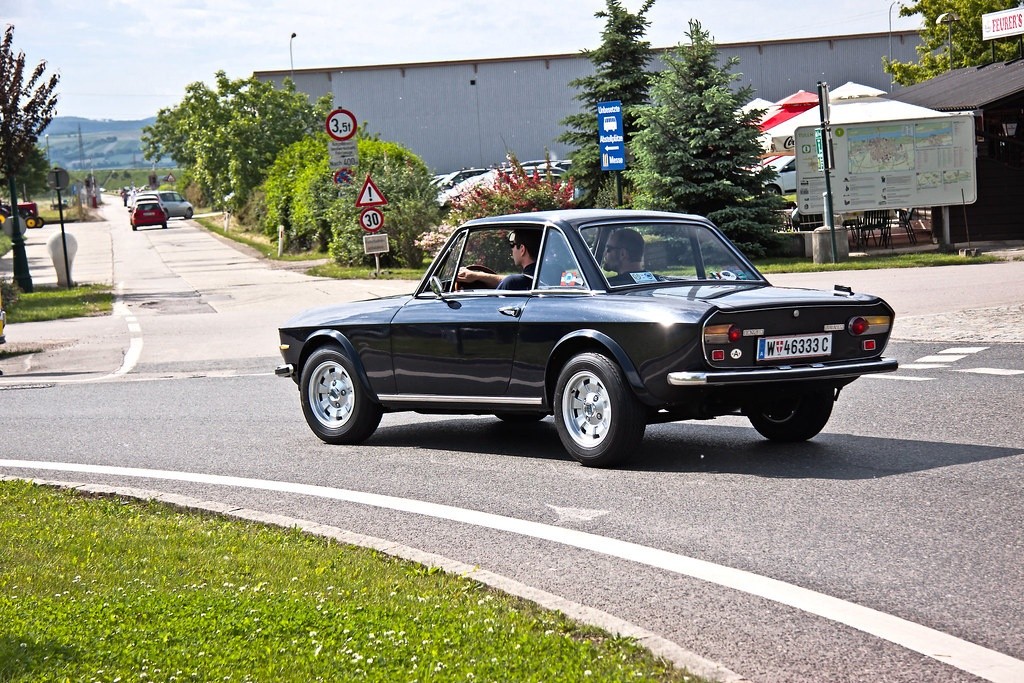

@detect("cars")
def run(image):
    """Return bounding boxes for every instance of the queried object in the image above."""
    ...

[273,208,899,466]
[130,200,168,230]
[132,197,162,213]
[425,159,583,208]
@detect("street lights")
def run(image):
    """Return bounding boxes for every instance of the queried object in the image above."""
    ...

[289,34,296,81]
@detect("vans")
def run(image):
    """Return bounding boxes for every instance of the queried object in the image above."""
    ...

[141,191,194,219]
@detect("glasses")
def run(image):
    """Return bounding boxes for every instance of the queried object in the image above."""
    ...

[509,241,515,248]
[605,245,621,252]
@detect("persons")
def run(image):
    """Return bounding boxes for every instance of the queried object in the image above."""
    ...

[603,228,668,286]
[455,228,562,297]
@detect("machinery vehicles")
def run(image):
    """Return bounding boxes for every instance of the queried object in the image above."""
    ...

[0,200,44,228]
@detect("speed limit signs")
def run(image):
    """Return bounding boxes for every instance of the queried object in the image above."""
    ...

[360,208,384,232]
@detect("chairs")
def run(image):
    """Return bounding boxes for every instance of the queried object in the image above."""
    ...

[841,207,920,253]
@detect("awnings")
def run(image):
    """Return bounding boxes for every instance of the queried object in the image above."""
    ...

[737,79,952,152]
